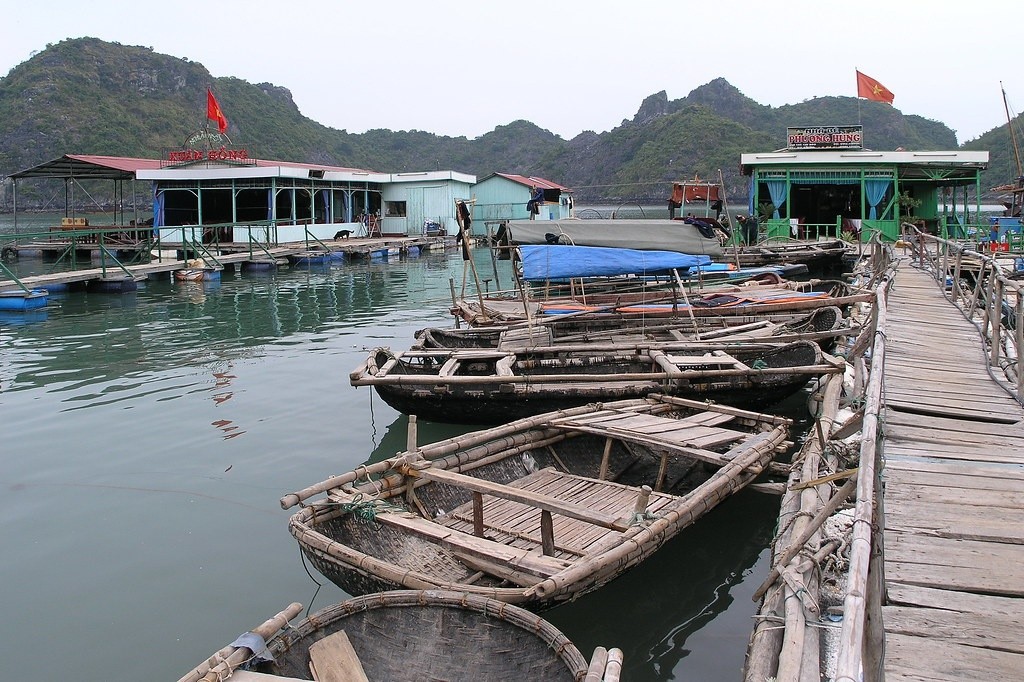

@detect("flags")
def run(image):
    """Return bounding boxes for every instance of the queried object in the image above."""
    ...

[207,88,228,133]
[856,70,895,105]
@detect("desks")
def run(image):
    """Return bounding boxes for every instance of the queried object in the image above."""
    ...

[48,223,154,243]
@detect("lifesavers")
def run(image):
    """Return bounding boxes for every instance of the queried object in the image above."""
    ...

[1,246,18,259]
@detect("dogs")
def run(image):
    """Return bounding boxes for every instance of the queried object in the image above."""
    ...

[333,230,355,242]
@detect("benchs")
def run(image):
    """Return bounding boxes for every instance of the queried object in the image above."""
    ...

[404,466,630,558]
[495,356,516,377]
[375,350,406,377]
[333,487,570,579]
[439,358,461,377]
[543,409,753,465]
[650,350,749,373]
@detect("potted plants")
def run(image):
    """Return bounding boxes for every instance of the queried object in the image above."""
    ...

[893,191,923,243]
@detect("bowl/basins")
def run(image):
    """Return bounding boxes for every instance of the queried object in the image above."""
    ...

[427,231,439,237]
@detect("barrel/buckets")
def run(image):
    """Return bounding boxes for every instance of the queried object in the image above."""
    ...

[1014,258,1024,274]
[424,221,440,234]
[439,230,447,236]
[976,240,1009,253]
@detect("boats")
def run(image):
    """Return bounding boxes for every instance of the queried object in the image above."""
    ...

[278,395,797,616]
[410,172,857,356]
[176,591,625,682]
[350,340,848,424]
